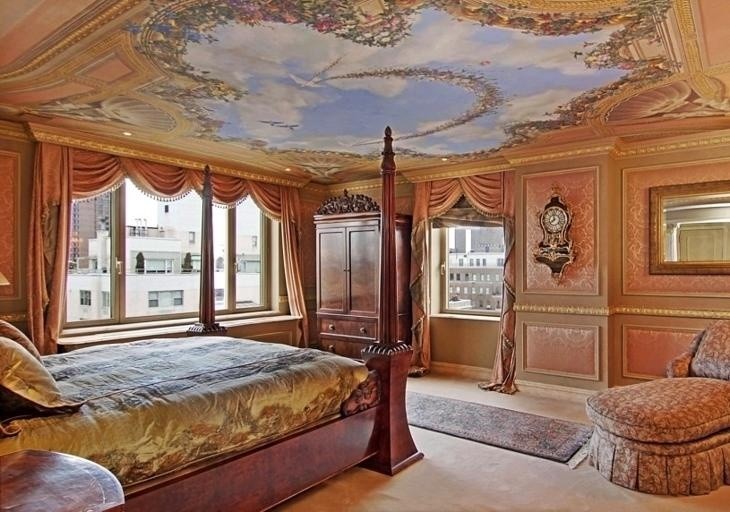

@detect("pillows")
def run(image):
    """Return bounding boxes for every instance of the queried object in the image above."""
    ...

[1,318,88,436]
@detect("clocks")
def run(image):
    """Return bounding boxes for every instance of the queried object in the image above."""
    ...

[533,195,578,287]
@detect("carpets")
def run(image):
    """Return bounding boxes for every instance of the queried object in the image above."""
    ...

[405,391,594,471]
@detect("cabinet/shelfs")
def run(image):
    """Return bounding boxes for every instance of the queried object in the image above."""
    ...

[311,188,413,359]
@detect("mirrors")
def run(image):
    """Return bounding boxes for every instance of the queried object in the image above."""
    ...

[649,179,730,275]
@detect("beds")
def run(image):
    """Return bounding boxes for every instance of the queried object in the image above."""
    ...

[2,336,424,511]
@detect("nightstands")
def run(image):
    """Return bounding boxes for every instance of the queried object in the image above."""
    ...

[0,449,125,512]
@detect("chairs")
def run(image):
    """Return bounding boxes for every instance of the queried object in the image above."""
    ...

[585,318,730,497]
